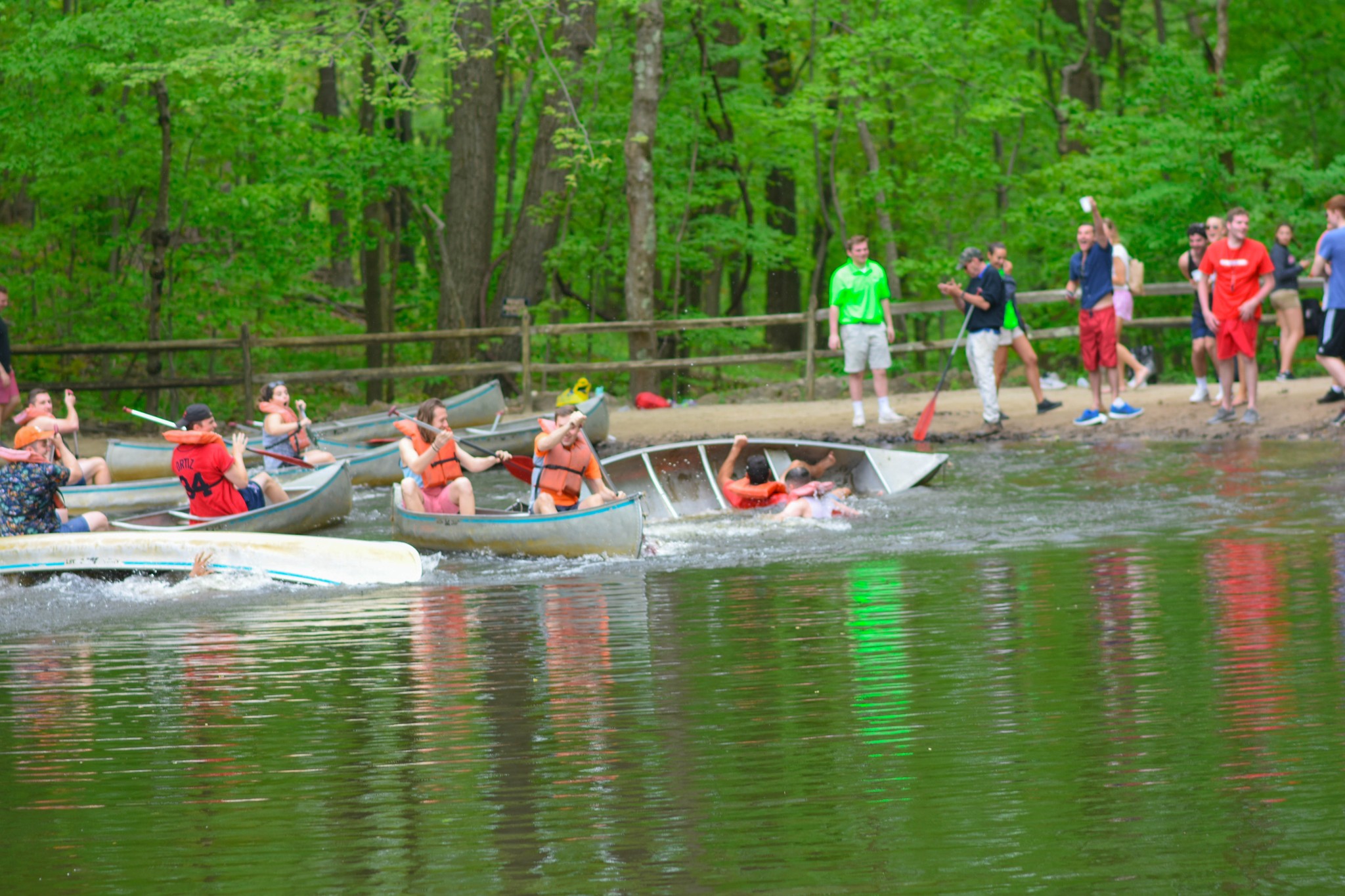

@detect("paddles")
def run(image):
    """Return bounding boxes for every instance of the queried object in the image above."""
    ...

[68,389,80,459]
[579,424,662,559]
[912,288,984,441]
[228,421,261,435]
[122,406,315,469]
[246,418,263,428]
[387,405,536,485]
[491,410,503,430]
[298,402,319,448]
[49,424,59,463]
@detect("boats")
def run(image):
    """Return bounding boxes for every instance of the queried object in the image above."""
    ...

[388,479,646,562]
[526,438,951,528]
[0,378,612,589]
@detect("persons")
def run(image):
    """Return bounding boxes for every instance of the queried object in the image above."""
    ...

[712,433,870,525]
[397,398,513,516]
[1309,194,1345,427]
[1198,207,1275,425]
[1063,196,1144,427]
[171,404,290,525]
[1097,217,1151,393]
[1267,222,1312,382]
[0,284,21,448]
[828,235,908,428]
[937,247,1006,437]
[0,423,111,540]
[13,387,111,486]
[1179,217,1249,406]
[181,549,214,583]
[528,404,626,515]
[257,381,336,470]
[984,241,1062,419]
[657,330,691,404]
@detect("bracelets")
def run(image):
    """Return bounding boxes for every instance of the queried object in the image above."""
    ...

[297,420,302,429]
[431,442,440,452]
[960,291,966,299]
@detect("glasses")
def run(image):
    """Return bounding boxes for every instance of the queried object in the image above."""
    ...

[268,380,285,387]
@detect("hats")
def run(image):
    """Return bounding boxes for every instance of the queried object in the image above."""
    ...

[13,426,55,449]
[175,404,214,428]
[956,247,984,270]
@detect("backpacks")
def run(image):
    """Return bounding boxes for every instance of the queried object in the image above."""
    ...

[1130,258,1144,295]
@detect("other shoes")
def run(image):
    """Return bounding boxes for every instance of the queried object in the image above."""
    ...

[1276,370,1297,381]
[1036,398,1062,414]
[1189,387,1210,403]
[1211,401,1224,406]
[1240,409,1262,425]
[877,411,910,424]
[851,414,866,428]
[977,419,1004,438]
[1216,388,1233,400]
[999,411,1010,420]
[1207,407,1236,424]
[1231,398,1249,405]
[1073,409,1107,426]
[1108,403,1144,420]
[1317,386,1345,404]
